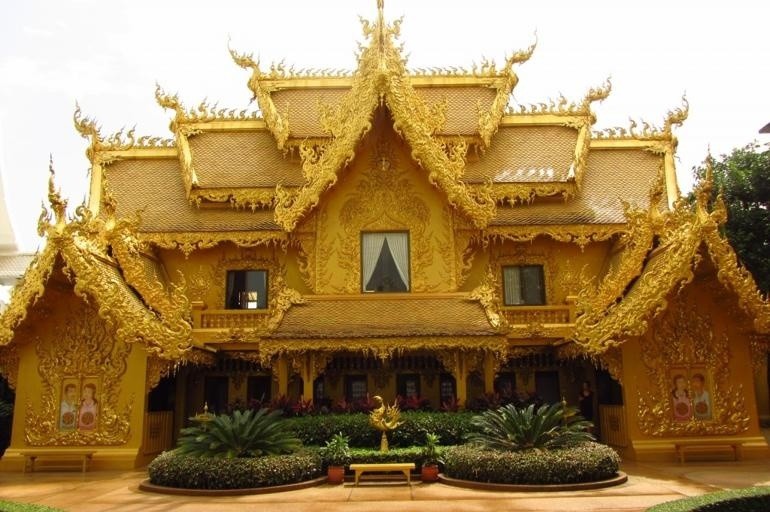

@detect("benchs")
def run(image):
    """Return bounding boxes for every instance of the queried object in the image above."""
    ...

[670,440,745,463]
[350,463,417,486]
[20,451,94,474]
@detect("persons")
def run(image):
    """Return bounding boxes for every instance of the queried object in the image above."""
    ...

[79,383,99,430]
[691,372,713,421]
[670,373,693,422]
[60,382,76,428]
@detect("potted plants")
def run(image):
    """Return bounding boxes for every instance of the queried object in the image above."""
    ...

[324,432,349,485]
[421,431,442,482]
